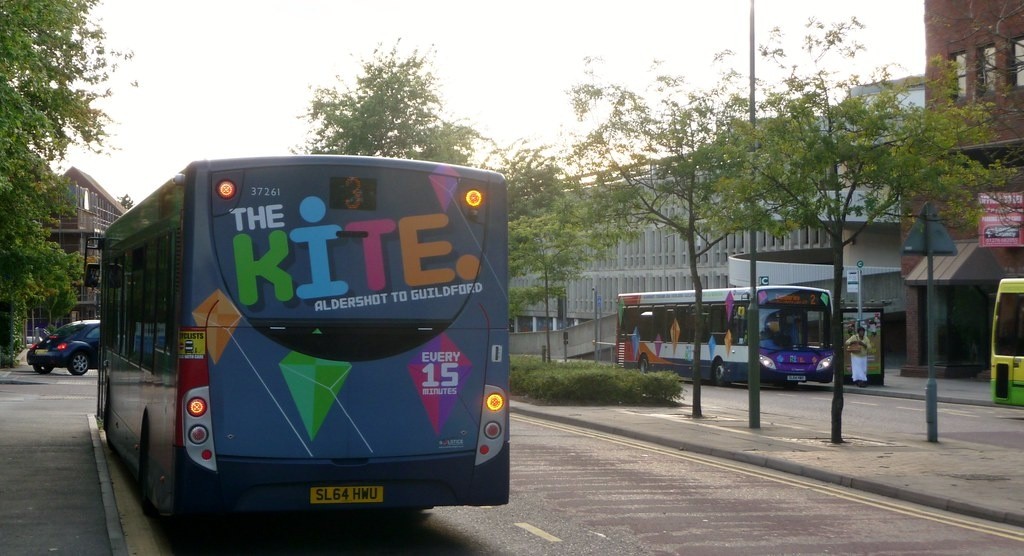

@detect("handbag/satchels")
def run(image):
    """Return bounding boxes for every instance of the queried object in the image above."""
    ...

[846,334,861,352]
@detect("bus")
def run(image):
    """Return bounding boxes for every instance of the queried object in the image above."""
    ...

[991,278,1024,407]
[615,286,860,386]
[85,155,511,523]
[27,330,50,344]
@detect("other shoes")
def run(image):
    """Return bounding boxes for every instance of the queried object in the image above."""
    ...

[855,380,868,388]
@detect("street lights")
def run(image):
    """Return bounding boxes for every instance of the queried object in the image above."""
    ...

[592,287,597,361]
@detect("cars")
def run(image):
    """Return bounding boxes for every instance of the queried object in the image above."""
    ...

[25,320,102,376]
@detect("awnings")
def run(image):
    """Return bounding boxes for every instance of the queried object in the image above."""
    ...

[904,238,1002,285]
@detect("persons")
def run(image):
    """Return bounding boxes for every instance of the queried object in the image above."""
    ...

[845,327,871,388]
